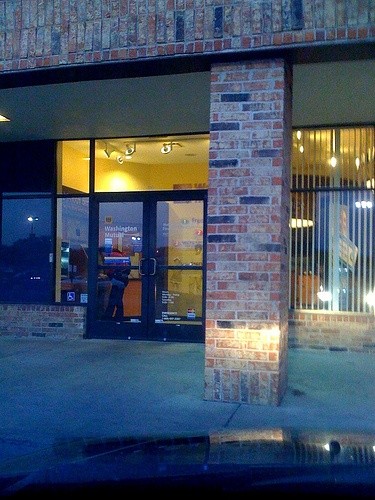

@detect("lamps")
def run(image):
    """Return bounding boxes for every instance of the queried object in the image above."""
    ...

[103,149,116,158]
[116,154,125,165]
[160,142,183,154]
[125,143,136,156]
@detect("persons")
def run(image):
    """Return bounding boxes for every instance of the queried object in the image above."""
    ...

[99,243,131,321]
